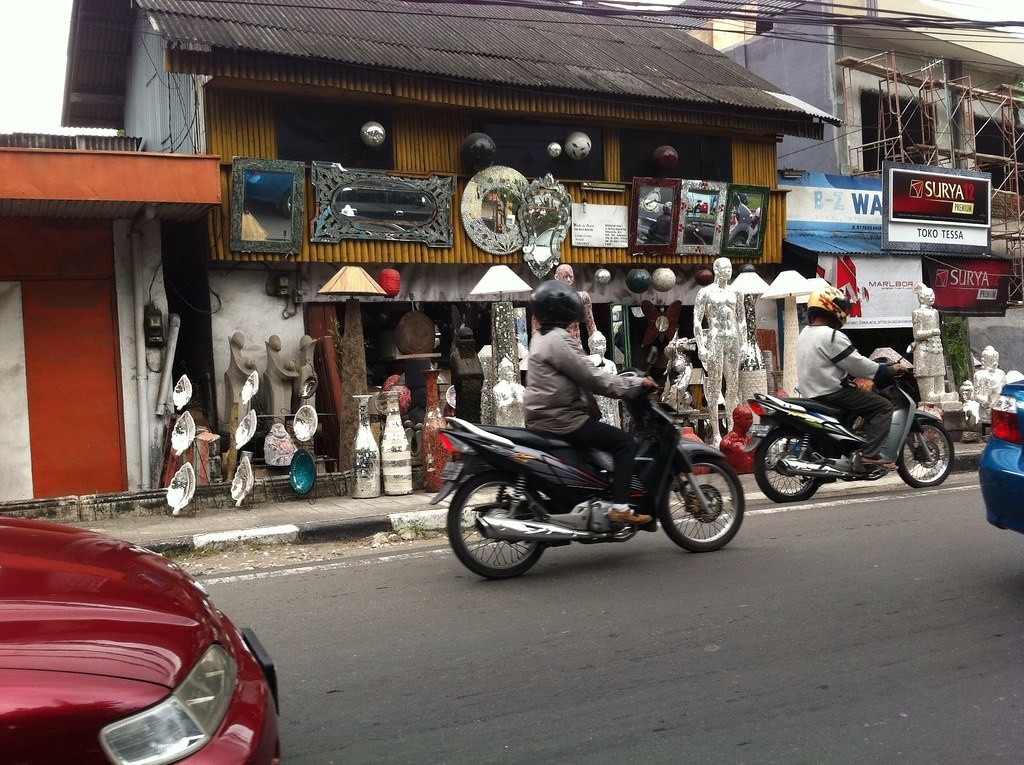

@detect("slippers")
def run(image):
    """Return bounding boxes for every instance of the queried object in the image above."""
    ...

[861,460,899,471]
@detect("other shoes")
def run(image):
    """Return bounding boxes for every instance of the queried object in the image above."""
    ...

[609,508,652,525]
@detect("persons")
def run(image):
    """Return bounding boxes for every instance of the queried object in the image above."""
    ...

[694,199,708,213]
[746,207,760,247]
[532,265,597,349]
[795,286,908,471]
[720,406,756,473]
[912,282,959,401]
[643,200,672,243]
[974,345,1006,423]
[694,257,747,449]
[493,357,526,429]
[588,331,621,429]
[524,280,659,523]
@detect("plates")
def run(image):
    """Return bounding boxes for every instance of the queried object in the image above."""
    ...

[300,376,317,399]
[289,449,316,494]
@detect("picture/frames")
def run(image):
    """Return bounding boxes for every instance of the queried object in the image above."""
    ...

[676,181,729,257]
[721,182,770,260]
[309,160,455,248]
[516,173,572,279]
[626,175,683,256]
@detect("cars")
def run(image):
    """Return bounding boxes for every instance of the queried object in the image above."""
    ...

[0,513,283,765]
[977,379,1024,536]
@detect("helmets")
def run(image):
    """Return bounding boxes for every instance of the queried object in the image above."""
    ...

[733,193,740,205]
[808,286,850,329]
[738,192,747,204]
[531,279,584,323]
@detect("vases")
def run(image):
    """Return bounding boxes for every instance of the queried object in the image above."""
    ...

[350,390,413,499]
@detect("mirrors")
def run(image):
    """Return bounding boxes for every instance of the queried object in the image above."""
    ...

[231,156,305,254]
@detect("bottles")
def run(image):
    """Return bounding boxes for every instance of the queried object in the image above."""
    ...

[292,405,317,441]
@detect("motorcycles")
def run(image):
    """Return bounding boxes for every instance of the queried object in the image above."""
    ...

[745,342,952,503]
[429,345,747,581]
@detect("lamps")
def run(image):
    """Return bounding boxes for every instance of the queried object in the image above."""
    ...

[467,265,534,305]
[317,263,387,300]
